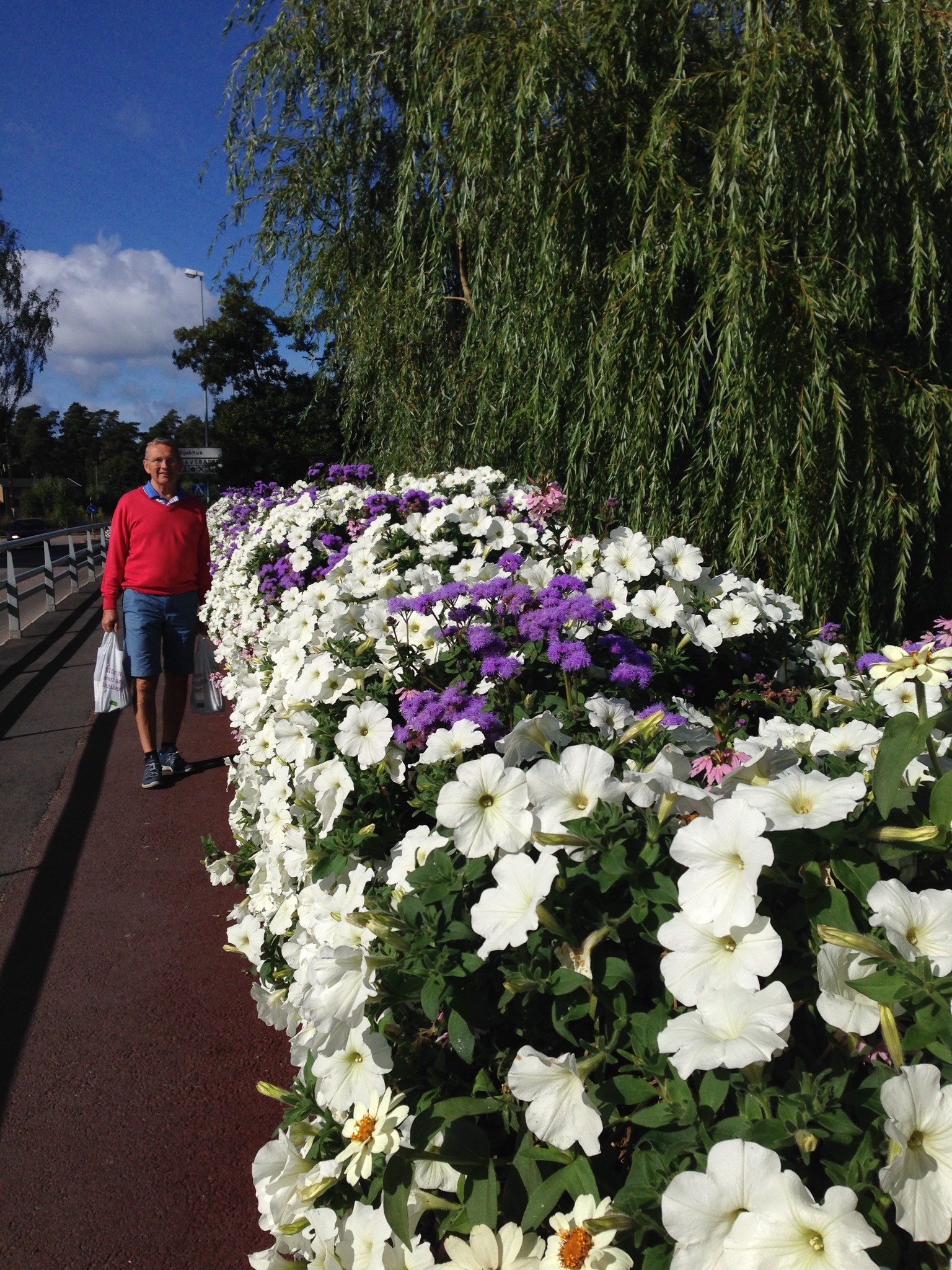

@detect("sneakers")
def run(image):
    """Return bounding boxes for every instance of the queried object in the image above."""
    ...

[158,747,194,773]
[142,755,164,788]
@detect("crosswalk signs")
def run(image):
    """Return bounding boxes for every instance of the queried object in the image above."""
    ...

[191,485,205,498]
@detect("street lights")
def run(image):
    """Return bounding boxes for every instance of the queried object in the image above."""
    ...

[185,269,210,509]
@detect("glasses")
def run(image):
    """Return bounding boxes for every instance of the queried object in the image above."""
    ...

[144,457,180,466]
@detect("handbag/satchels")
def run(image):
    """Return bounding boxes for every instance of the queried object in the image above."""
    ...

[93,630,132,714]
[190,632,225,715]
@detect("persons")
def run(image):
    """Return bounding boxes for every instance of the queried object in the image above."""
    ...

[101,438,211,788]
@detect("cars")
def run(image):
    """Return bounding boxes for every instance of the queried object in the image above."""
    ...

[103,526,111,539]
[7,518,51,548]
